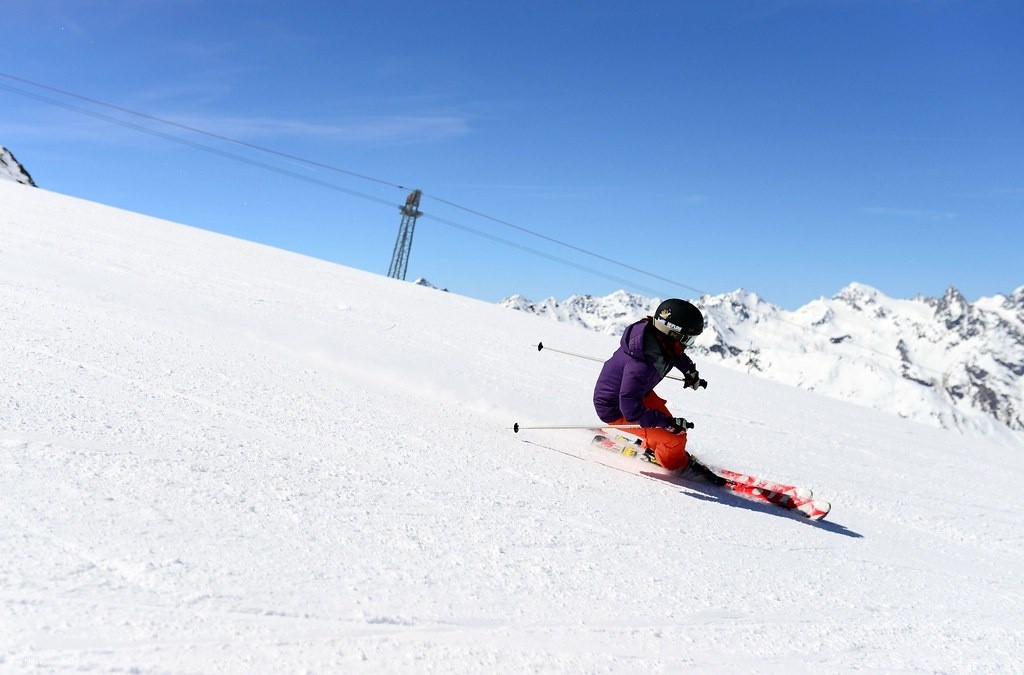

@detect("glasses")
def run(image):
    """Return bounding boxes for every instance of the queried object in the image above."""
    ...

[653,316,698,347]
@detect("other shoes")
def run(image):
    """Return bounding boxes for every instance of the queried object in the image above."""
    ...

[682,460,712,481]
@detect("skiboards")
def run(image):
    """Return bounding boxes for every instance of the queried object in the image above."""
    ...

[587,424,831,520]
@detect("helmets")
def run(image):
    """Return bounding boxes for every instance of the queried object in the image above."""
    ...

[654,299,703,344]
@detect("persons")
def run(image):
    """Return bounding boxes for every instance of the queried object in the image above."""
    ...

[593,299,722,486]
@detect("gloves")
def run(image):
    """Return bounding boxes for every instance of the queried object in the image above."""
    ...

[664,418,688,435]
[684,364,700,391]
